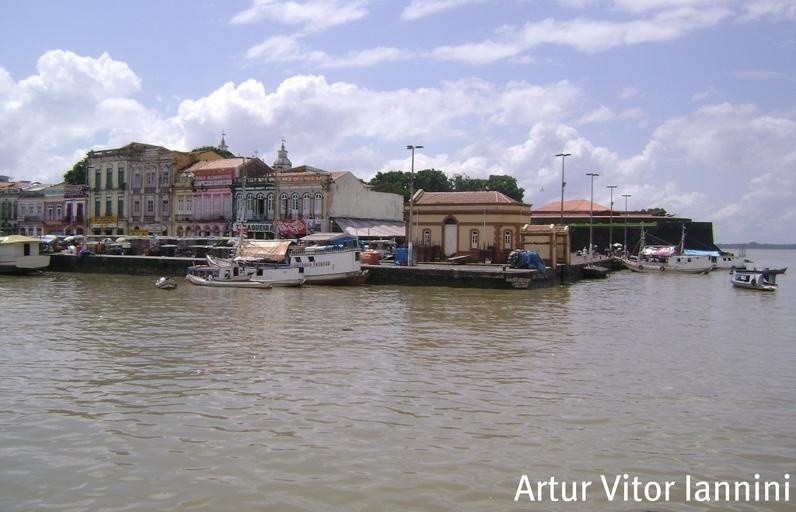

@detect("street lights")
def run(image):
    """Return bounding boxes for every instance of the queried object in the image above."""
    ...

[555,153,571,224]
[406,145,424,267]
[585,173,632,267]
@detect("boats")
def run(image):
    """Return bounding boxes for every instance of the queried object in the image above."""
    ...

[154,276,178,289]
[729,266,788,291]
[0,234,51,276]
[185,238,363,289]
[614,223,747,273]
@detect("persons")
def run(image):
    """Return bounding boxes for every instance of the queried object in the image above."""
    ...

[159,275,165,283]
[208,272,214,280]
[165,274,170,281]
[582,244,632,261]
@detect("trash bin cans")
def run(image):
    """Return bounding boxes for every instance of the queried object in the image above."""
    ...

[396,248,408,265]
